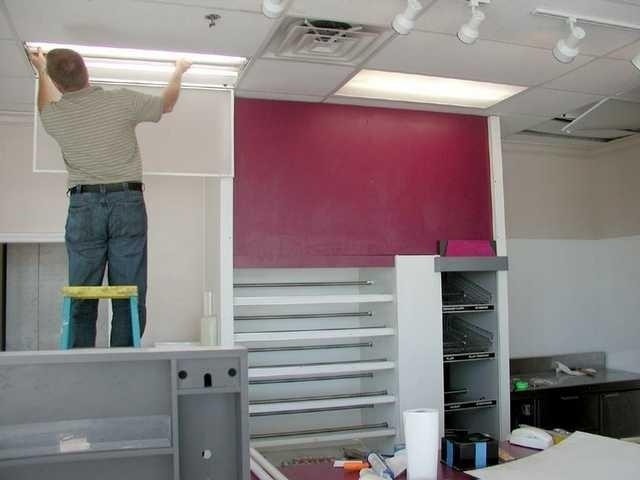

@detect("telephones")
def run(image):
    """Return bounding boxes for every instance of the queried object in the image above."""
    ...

[510,427,554,449]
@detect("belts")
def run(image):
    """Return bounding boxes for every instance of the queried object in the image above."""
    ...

[70,180,142,194]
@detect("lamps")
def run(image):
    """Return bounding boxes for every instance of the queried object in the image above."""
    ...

[262,0,287,19]
[391,0,586,65]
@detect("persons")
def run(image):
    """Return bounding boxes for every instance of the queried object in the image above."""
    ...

[27,45,193,349]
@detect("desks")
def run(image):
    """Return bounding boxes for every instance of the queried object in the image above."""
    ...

[249,426,640,480]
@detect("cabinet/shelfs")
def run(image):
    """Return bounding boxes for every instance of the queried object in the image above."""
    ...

[508,380,640,440]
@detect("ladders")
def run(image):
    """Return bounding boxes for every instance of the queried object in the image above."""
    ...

[61,286,141,350]
